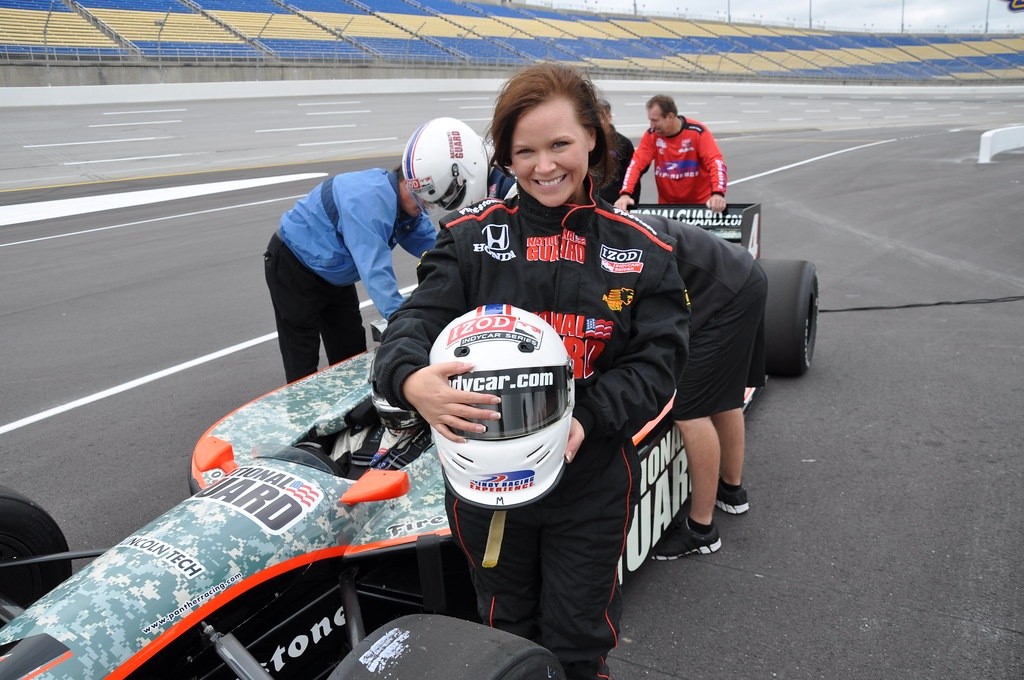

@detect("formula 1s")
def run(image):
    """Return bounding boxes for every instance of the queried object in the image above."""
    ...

[1,205,820,679]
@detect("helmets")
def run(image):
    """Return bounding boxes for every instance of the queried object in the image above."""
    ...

[368,316,425,432]
[428,303,574,509]
[402,117,489,216]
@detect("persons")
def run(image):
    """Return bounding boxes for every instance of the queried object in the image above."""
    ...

[262,159,437,386]
[367,60,769,680]
[613,94,728,212]
[596,98,641,210]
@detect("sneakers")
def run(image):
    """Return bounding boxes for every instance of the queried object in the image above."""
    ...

[648,517,721,560]
[714,479,750,514]
